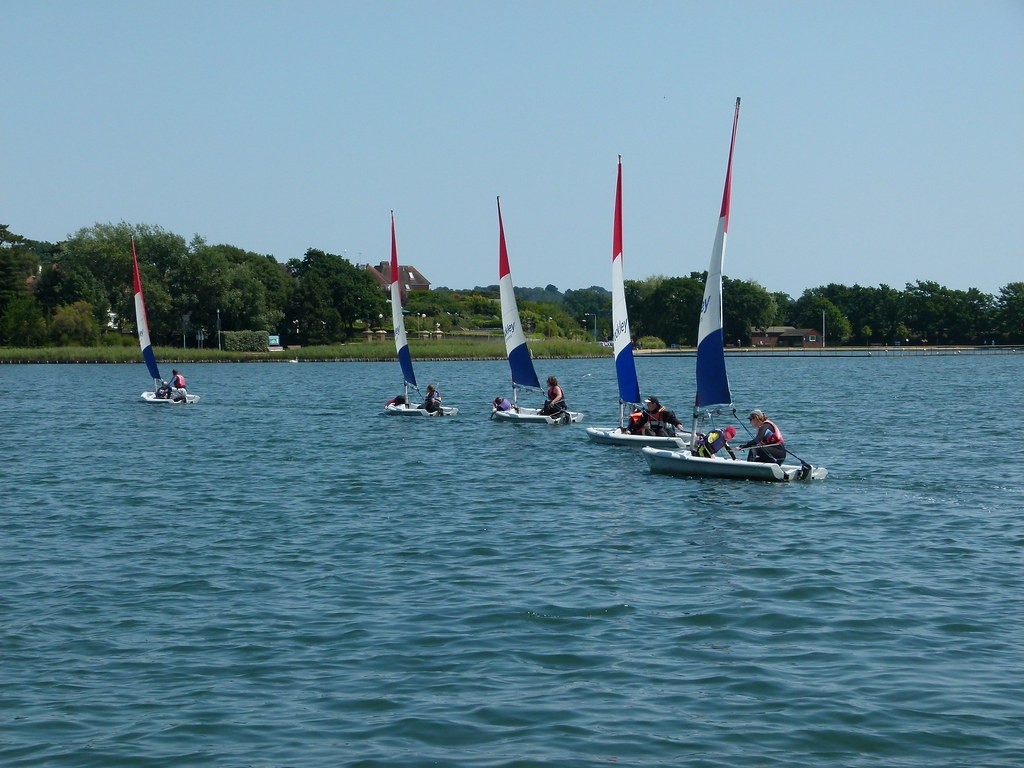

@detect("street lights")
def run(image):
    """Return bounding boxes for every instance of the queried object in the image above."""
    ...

[549,317,552,340]
[417,313,420,338]
[379,314,383,330]
[584,313,596,342]
[422,313,426,331]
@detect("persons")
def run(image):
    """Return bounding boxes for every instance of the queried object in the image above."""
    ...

[386,395,405,407]
[984,339,987,345]
[155,379,172,399]
[620,395,684,438]
[417,384,441,412]
[546,375,567,419]
[737,338,741,348]
[691,425,736,457]
[991,337,994,345]
[735,410,786,465]
[493,397,511,411]
[163,368,188,400]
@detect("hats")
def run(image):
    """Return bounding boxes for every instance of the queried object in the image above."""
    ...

[725,426,735,441]
[746,410,763,420]
[643,396,658,403]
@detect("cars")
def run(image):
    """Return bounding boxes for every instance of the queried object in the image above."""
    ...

[595,340,634,350]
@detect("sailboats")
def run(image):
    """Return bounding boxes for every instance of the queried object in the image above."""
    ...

[642,96,815,482]
[132,235,200,405]
[585,155,705,449]
[385,210,459,416]
[492,196,584,424]
